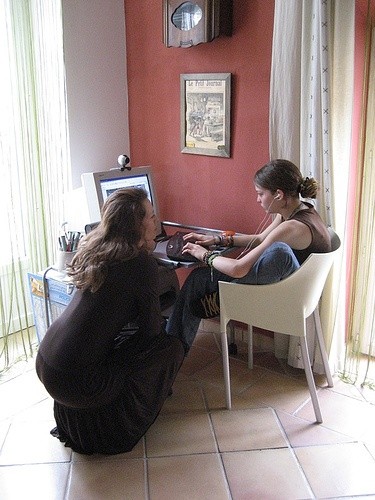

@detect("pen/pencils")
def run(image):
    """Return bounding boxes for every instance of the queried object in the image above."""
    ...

[58,231,83,252]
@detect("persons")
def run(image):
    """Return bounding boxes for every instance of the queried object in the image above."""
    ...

[35,186,185,456]
[165,159,332,397]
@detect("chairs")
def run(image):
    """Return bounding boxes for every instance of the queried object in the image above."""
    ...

[220,227,344,422]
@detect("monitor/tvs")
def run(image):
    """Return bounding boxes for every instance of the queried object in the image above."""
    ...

[83,165,162,239]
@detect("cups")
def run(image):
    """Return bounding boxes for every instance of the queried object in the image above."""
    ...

[55,251,75,271]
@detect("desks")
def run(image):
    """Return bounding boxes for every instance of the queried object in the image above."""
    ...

[28,239,238,345]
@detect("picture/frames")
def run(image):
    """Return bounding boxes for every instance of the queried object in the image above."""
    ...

[180,72,233,157]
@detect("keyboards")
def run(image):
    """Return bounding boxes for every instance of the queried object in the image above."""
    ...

[165,230,210,262]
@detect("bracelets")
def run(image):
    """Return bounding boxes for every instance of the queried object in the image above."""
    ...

[213,234,234,248]
[202,251,222,267]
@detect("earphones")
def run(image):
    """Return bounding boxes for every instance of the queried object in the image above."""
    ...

[275,193,280,199]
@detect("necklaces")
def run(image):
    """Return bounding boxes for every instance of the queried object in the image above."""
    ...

[287,202,303,218]
